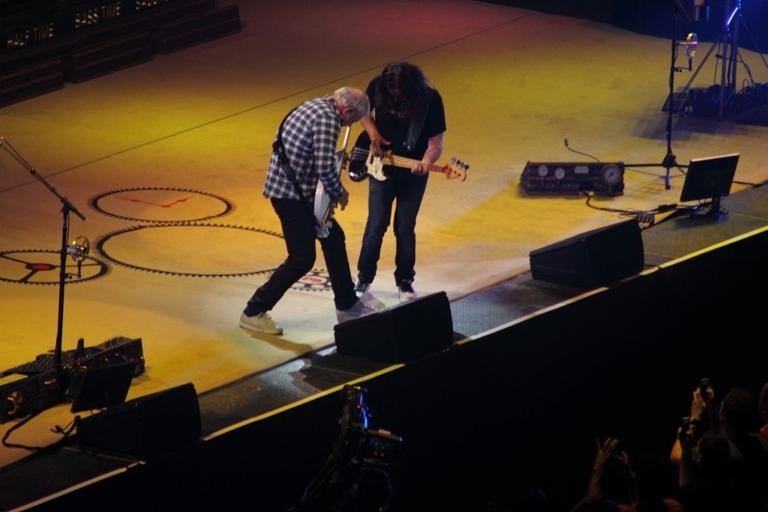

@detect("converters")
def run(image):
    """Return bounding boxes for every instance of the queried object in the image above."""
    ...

[660,203,677,212]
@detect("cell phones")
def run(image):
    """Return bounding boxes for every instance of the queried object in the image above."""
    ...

[686,424,696,443]
[700,378,709,401]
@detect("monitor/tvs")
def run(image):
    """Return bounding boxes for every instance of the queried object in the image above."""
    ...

[681,151,739,215]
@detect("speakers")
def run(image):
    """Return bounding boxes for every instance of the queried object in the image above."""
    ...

[530,216,645,286]
[75,383,202,456]
[332,292,453,363]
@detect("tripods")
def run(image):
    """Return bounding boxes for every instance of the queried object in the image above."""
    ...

[625,0,692,190]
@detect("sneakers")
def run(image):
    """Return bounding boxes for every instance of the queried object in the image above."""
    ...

[239,312,283,335]
[398,280,416,303]
[353,280,372,301]
[337,301,380,317]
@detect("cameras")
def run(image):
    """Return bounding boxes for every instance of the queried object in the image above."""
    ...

[611,452,623,461]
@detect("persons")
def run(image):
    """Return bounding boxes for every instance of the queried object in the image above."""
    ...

[569,375,768,512]
[354,61,446,305]
[239,87,378,335]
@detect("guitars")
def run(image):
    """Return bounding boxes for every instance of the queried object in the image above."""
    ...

[349,130,469,182]
[314,125,351,238]
